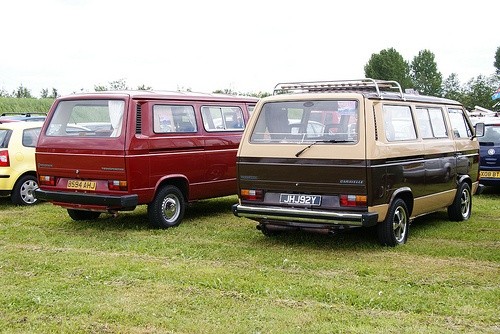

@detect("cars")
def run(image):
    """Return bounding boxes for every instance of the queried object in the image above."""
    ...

[0,112,90,206]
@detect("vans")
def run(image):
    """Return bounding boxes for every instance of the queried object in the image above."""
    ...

[232,78,481,247]
[35,91,262,229]
